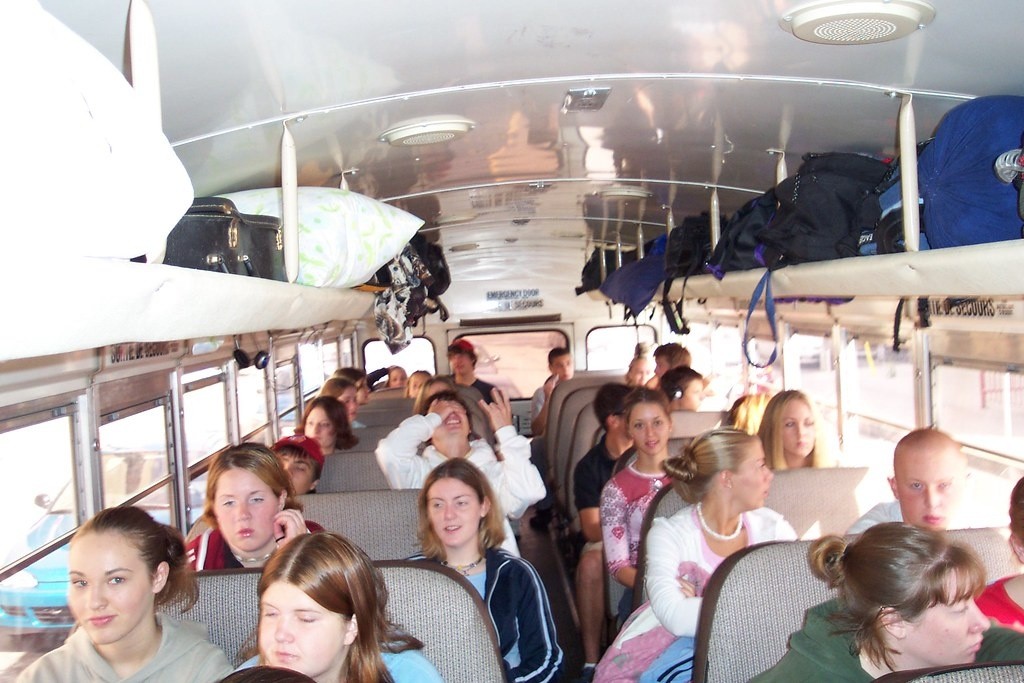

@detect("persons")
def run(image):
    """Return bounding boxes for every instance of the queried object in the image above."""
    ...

[449,339,501,403]
[375,370,549,563]
[569,382,828,683]
[747,522,991,683]
[975,477,1024,634]
[366,365,408,390]
[178,434,327,574]
[15,507,232,682]
[626,340,713,412]
[298,368,370,455]
[840,427,971,537]
[530,347,576,531]
[219,531,444,682]
[400,457,564,683]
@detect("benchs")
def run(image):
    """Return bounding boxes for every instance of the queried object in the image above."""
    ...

[155,379,507,683]
[543,369,1024,683]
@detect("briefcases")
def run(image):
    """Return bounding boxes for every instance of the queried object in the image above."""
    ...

[167,196,288,280]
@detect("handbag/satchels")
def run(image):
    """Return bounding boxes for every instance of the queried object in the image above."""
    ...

[574,94,1023,370]
[375,236,450,301]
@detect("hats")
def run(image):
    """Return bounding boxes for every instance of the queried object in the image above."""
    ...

[451,339,475,356]
[269,434,323,473]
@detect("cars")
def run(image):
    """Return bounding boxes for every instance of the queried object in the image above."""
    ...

[1,446,215,649]
[322,338,520,398]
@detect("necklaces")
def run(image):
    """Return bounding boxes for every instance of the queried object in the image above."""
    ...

[440,557,483,578]
[635,462,667,492]
[697,502,743,540]
[232,540,279,563]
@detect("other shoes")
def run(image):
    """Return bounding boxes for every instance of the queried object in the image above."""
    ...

[530,509,553,529]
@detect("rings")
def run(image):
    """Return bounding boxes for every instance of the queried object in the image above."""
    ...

[274,536,285,542]
